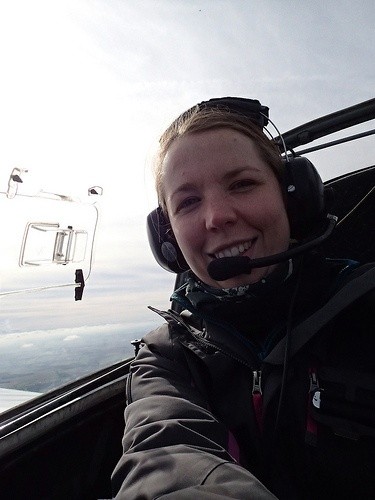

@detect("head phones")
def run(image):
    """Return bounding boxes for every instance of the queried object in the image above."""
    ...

[147,97,326,273]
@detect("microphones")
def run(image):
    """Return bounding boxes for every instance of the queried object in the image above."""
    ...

[208,214,338,281]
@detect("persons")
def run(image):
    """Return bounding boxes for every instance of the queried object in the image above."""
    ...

[109,96,374,499]
